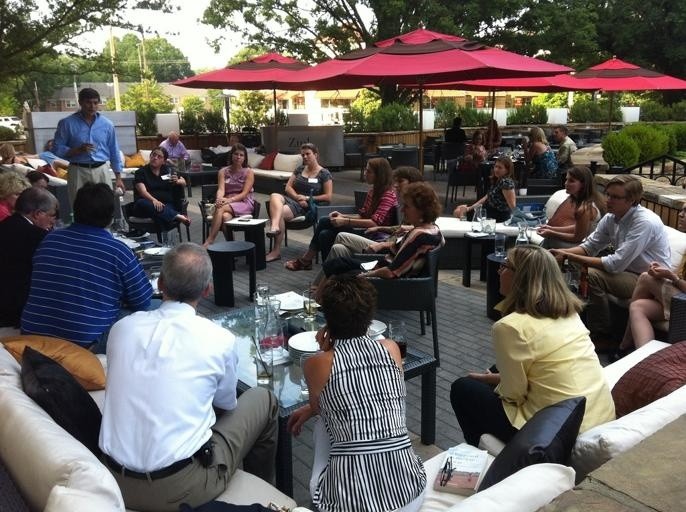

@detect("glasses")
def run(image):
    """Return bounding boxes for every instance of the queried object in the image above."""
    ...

[498,260,516,274]
[438,456,456,488]
[603,192,629,201]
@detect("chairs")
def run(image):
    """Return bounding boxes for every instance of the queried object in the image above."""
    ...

[525,174,563,185]
[265,183,318,254]
[423,136,441,182]
[344,139,363,167]
[339,234,447,367]
[443,160,483,206]
[439,140,462,166]
[314,189,402,233]
[196,186,261,246]
[120,180,191,245]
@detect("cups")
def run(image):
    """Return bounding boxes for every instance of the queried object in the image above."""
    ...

[170,167,177,182]
[300,353,316,396]
[256,282,270,300]
[303,290,320,321]
[149,266,163,296]
[255,344,274,379]
[389,321,407,366]
[494,231,506,257]
[460,206,467,221]
[253,293,268,324]
[481,208,487,218]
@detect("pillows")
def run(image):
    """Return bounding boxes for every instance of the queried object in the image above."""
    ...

[259,150,279,170]
[610,340,686,419]
[39,164,56,178]
[125,154,146,168]
[1,382,132,512]
[0,335,107,389]
[17,345,103,451]
[248,152,265,167]
[569,381,686,481]
[43,484,119,512]
[471,396,589,495]
[657,225,686,279]
[431,464,576,512]
[273,153,303,170]
[58,167,69,179]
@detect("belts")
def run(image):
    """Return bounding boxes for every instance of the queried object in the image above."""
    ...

[95,446,200,481]
[69,159,110,168]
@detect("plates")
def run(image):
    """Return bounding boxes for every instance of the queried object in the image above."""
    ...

[288,330,325,364]
[367,319,387,340]
[144,247,170,260]
[115,236,141,250]
[273,294,311,318]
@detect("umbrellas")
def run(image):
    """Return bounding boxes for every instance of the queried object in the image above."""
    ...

[574,54,686,131]
[284,29,576,172]
[402,66,599,131]
[168,53,315,137]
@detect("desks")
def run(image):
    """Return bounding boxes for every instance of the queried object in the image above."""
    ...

[204,240,257,304]
[222,218,269,273]
[177,170,218,198]
[461,229,507,287]
[482,249,509,321]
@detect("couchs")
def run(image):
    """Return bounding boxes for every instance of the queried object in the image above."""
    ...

[249,152,305,195]
[430,189,573,269]
[600,212,686,343]
[294,336,686,510]
[140,150,225,186]
[1,324,299,510]
[0,149,141,189]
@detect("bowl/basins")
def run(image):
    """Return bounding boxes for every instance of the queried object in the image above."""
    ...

[254,319,289,361]
[482,218,496,234]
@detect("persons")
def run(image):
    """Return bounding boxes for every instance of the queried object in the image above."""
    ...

[52,88,126,222]
[288,275,427,512]
[553,124,578,173]
[266,142,333,263]
[450,244,616,444]
[548,175,672,355]
[309,165,426,295]
[536,167,605,248]
[453,155,516,220]
[606,196,686,363]
[202,143,255,248]
[461,130,487,172]
[521,127,558,178]
[0,186,59,328]
[444,117,485,140]
[131,147,191,242]
[308,183,444,305]
[159,131,189,174]
[96,242,279,512]
[484,120,501,147]
[284,158,400,271]
[213,135,239,167]
[20,181,162,355]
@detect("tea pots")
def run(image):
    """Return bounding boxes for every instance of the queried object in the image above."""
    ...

[264,300,284,360]
[515,222,529,247]
[471,204,482,232]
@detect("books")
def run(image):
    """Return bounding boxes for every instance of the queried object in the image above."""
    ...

[434,447,488,496]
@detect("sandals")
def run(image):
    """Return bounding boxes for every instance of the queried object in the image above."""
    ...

[266,255,282,262]
[265,229,282,239]
[284,258,313,272]
[184,215,193,228]
